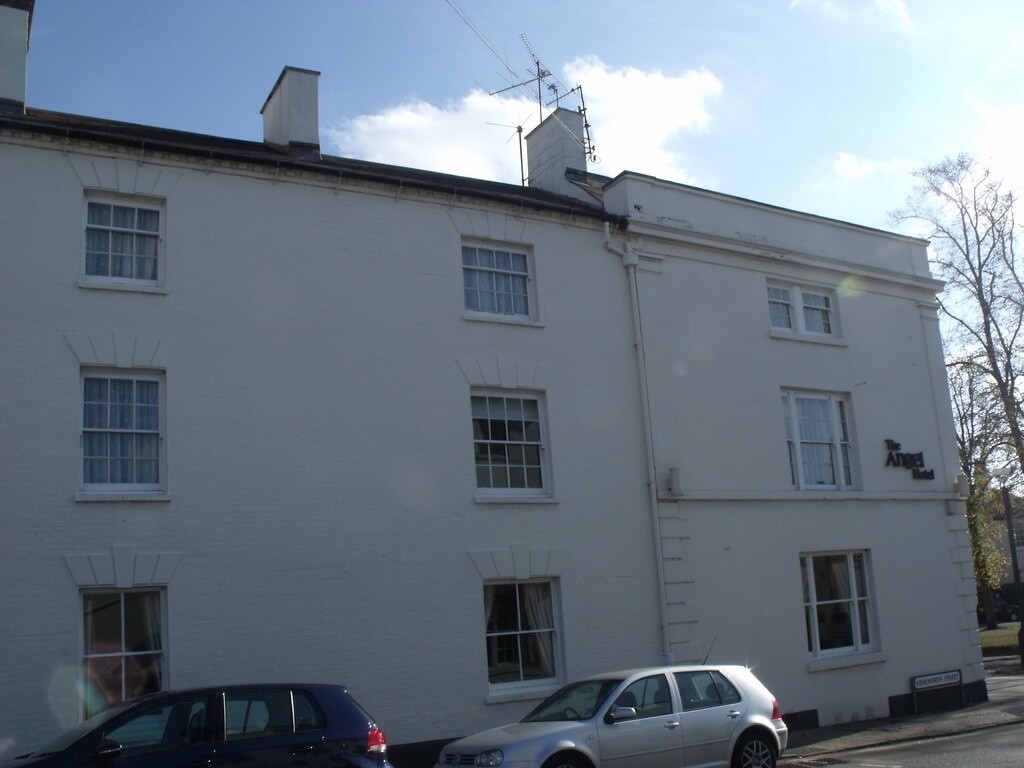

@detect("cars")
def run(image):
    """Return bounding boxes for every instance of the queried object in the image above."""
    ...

[435,666,788,768]
[1,679,393,768]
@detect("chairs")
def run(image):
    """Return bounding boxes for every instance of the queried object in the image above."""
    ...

[697,682,730,706]
[612,692,642,716]
